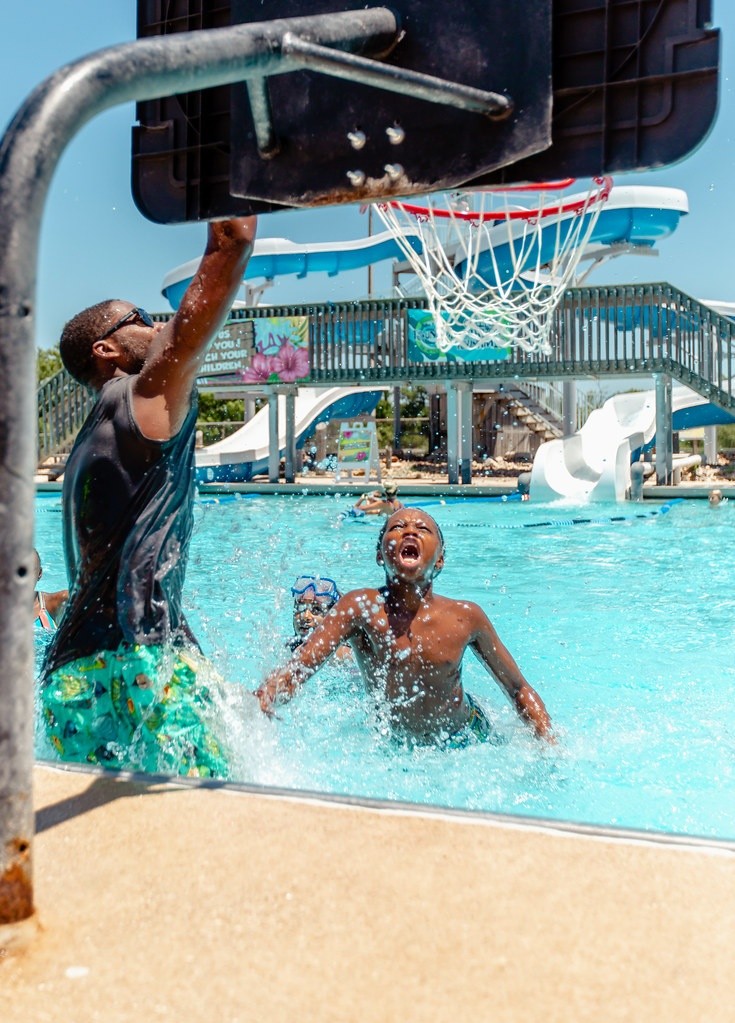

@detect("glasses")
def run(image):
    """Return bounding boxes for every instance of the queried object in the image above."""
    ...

[290,574,341,601]
[100,306,154,341]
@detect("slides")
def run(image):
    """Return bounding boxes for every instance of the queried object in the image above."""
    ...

[162,223,433,485]
[454,184,735,504]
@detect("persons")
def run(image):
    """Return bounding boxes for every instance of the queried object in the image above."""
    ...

[253,507,550,749]
[287,575,354,668]
[354,481,404,515]
[40,215,257,781]
[33,547,69,629]
[708,489,723,504]
